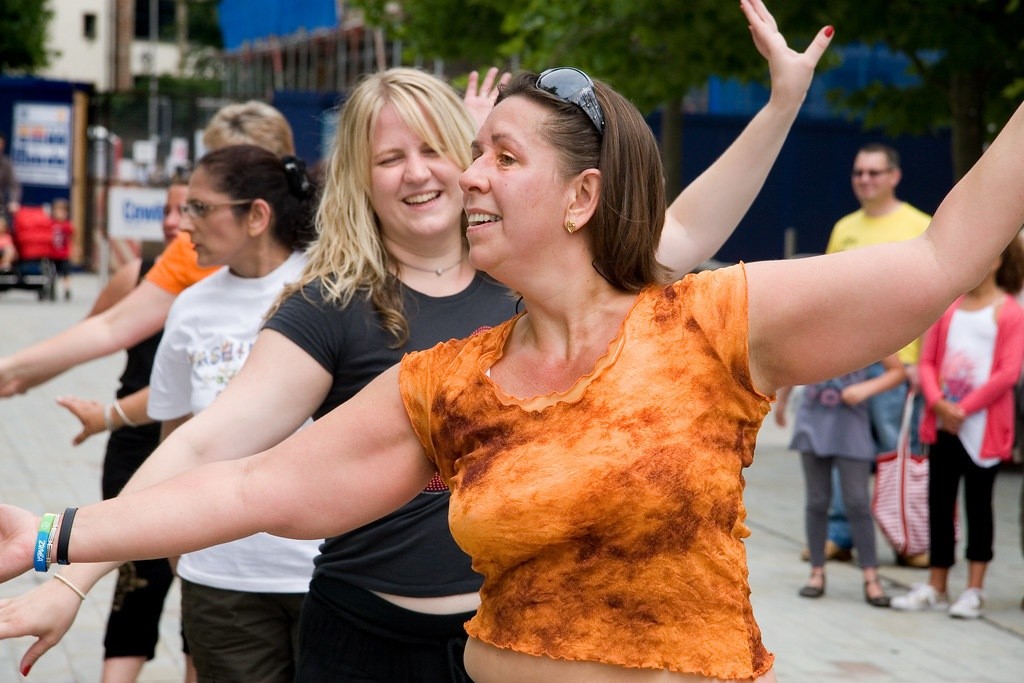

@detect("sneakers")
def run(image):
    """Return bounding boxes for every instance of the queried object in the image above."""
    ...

[948,590,986,618]
[890,586,950,610]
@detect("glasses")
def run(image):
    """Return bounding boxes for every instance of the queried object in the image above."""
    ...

[851,169,893,177]
[536,67,605,137]
[177,199,252,218]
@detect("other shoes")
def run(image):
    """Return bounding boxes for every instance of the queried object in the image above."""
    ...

[863,577,891,607]
[800,573,826,597]
[802,539,851,562]
[896,552,931,568]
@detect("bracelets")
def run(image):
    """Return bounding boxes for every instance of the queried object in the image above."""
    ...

[50,570,87,602]
[56,503,79,569]
[99,401,115,431]
[113,396,136,430]
[32,511,61,573]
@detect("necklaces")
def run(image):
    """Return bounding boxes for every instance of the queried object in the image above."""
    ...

[383,245,473,276]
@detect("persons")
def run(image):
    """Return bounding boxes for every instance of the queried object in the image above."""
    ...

[759,341,908,610]
[0,57,1024,680]
[139,65,517,679]
[1,144,199,681]
[0,0,828,679]
[1,147,24,273]
[791,144,947,569]
[54,379,150,454]
[883,242,1024,621]
[41,197,77,300]
[0,99,295,406]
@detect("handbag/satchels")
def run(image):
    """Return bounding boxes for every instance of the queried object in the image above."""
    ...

[872,387,960,556]
